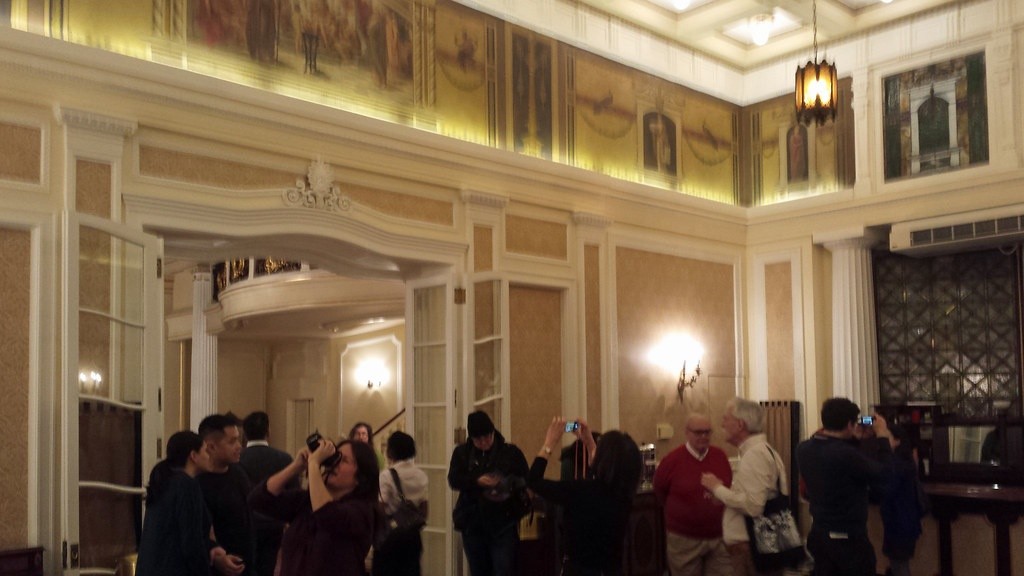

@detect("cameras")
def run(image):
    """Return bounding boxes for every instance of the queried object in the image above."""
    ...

[307,433,342,468]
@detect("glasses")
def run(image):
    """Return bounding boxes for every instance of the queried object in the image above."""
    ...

[689,428,713,436]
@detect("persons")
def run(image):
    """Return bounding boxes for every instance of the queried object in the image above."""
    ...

[135,412,299,576]
[798,400,922,576]
[651,415,735,576]
[247,440,379,576]
[702,399,786,576]
[448,412,530,576]
[528,417,643,576]
[370,431,428,576]
[350,421,384,470]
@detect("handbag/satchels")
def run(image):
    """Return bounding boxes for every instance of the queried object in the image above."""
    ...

[744,494,807,573]
[378,468,426,537]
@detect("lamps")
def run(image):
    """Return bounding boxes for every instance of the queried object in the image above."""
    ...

[793,0,838,127]
[676,361,702,405]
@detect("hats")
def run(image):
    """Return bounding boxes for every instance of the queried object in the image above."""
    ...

[467,411,496,436]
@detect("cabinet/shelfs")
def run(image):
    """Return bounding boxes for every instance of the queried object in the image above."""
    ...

[874,404,949,482]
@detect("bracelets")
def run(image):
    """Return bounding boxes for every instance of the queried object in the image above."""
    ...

[540,446,551,455]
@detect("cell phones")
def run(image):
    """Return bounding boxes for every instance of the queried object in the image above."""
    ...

[566,422,579,432]
[861,416,875,424]
